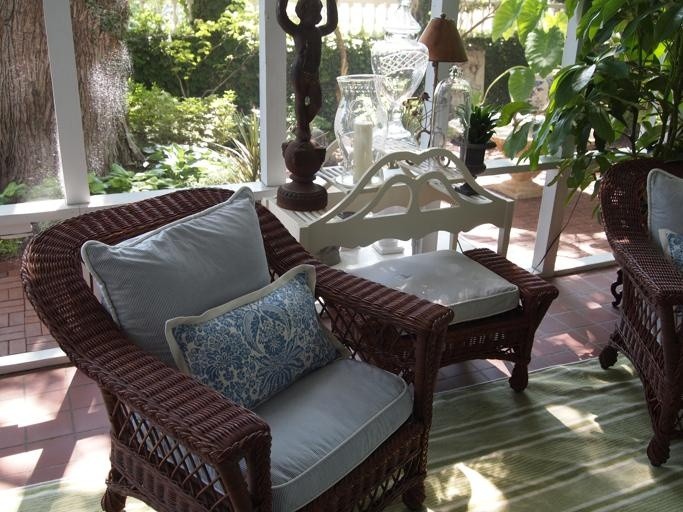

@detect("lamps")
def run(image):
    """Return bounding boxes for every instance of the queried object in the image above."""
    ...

[405,12,469,171]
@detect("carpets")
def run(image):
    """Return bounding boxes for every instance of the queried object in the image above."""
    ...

[2,351,682,511]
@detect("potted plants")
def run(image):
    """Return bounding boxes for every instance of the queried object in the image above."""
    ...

[450,102,497,197]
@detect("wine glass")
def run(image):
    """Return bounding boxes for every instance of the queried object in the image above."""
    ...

[370,37,429,141]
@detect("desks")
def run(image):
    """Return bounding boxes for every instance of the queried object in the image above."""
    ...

[262,169,516,320]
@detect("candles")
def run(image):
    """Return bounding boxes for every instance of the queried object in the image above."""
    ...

[352,121,374,186]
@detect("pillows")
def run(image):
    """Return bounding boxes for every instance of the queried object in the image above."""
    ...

[645,167,683,334]
[78,189,270,365]
[168,261,341,412]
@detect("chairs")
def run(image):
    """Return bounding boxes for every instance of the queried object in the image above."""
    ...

[18,187,455,512]
[596,152,683,469]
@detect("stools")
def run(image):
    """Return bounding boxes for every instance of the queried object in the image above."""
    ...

[342,249,561,393]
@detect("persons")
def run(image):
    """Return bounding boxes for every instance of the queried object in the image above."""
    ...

[275,0,339,141]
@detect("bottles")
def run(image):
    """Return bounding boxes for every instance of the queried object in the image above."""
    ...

[335,74,386,189]
[433,63,471,182]
